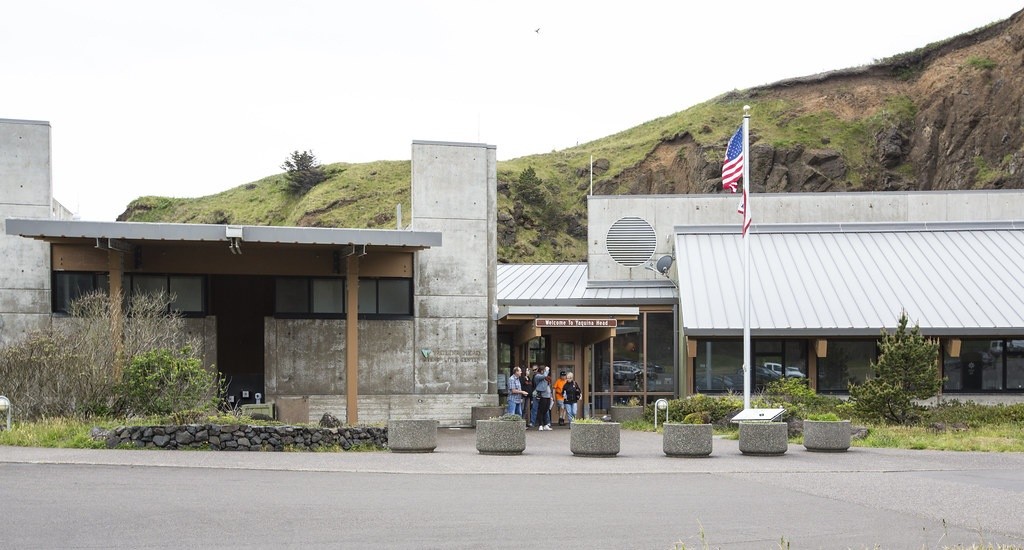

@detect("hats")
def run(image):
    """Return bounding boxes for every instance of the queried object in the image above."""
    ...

[560,371,566,376]
[537,366,545,372]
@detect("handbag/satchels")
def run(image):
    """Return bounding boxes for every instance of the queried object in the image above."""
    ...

[574,381,582,400]
[532,389,538,398]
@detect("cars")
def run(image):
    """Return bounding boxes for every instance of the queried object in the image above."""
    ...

[639,362,652,379]
[695,375,744,397]
[600,360,623,389]
[653,362,665,373]
[736,365,787,391]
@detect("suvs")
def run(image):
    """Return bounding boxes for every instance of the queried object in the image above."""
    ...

[609,361,644,382]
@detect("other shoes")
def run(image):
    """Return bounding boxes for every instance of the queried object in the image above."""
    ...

[539,426,543,431]
[544,425,552,431]
[527,423,535,428]
[559,418,566,426]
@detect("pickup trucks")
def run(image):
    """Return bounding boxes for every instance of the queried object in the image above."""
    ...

[764,362,804,381]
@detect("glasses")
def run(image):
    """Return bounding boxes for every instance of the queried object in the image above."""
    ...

[533,368,538,371]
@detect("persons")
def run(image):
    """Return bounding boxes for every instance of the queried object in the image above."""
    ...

[508,365,581,430]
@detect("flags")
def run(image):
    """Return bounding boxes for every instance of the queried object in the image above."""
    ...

[722,123,752,237]
